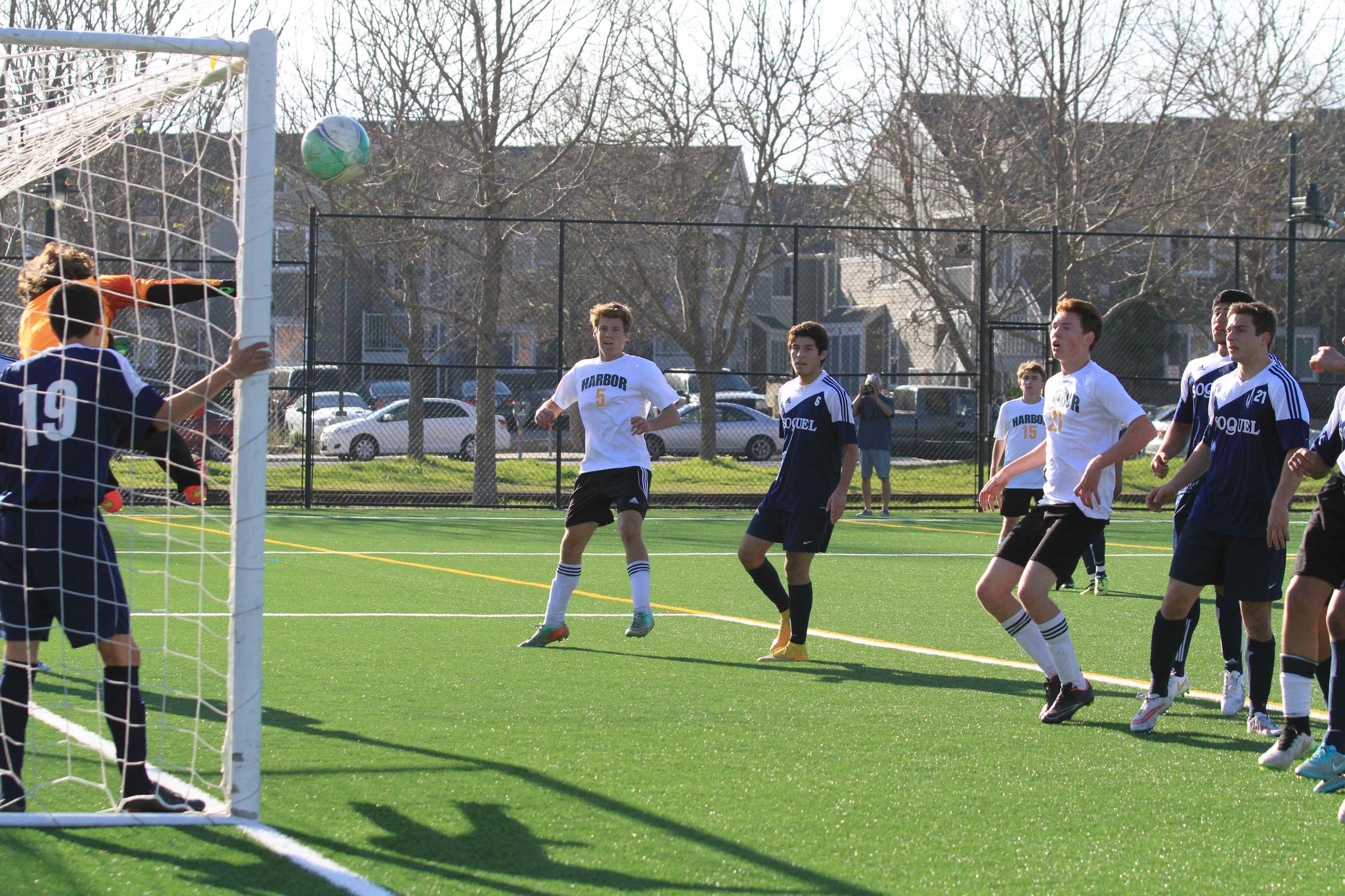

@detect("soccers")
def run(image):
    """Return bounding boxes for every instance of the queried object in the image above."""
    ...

[300,114,370,185]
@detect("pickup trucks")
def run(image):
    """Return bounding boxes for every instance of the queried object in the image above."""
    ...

[853,385,1002,457]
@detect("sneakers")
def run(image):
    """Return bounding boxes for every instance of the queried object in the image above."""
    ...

[1039,667,1082,718]
[1080,581,1095,595]
[102,489,123,512]
[1130,685,1174,732]
[757,641,808,662]
[1061,577,1074,589]
[770,619,791,652]
[1169,668,1190,699]
[855,509,872,516]
[1220,667,1245,716]
[1041,679,1095,724]
[880,510,890,516]
[0,794,26,812]
[1094,575,1109,595]
[1313,773,1345,793]
[517,621,569,647]
[183,454,208,502]
[625,611,654,637]
[1247,712,1284,736]
[1294,743,1345,778]
[118,781,205,813]
[1258,726,1316,771]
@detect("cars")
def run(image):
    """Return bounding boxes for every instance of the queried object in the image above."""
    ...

[320,397,511,462]
[285,390,376,446]
[456,380,517,428]
[643,366,785,461]
[356,379,412,412]
[155,384,235,461]
[1121,404,1324,459]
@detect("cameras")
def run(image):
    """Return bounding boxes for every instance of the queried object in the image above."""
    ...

[864,385,874,394]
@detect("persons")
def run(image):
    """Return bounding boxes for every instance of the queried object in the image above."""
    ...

[18,239,236,361]
[976,297,1158,724]
[1130,300,1310,737]
[989,359,1047,554]
[1081,420,1122,594]
[517,301,681,648]
[852,372,895,517]
[1150,289,1257,717]
[737,320,859,662]
[1258,336,1345,824]
[0,296,273,813]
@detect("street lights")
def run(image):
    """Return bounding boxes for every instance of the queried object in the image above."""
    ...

[29,92,80,251]
[1286,132,1337,379]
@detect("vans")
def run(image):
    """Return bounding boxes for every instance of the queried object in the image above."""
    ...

[267,365,345,424]
[520,368,572,416]
